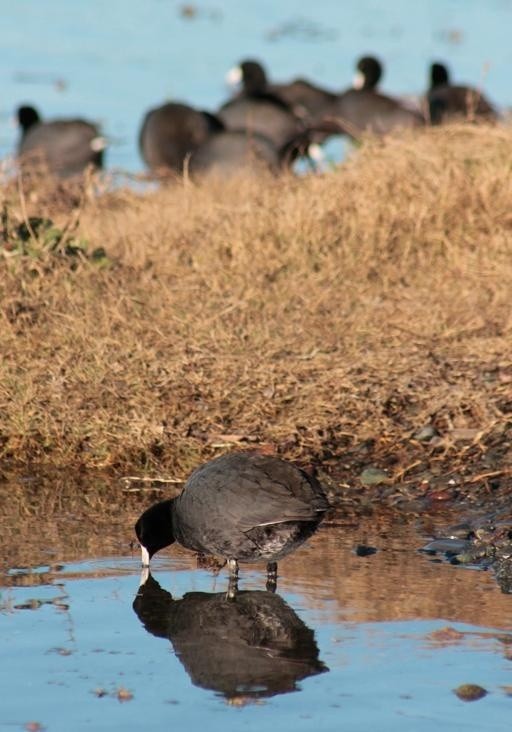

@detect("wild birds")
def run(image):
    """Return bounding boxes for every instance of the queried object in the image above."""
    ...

[137,50,501,192]
[132,449,334,577]
[120,570,329,706]
[10,103,108,189]
